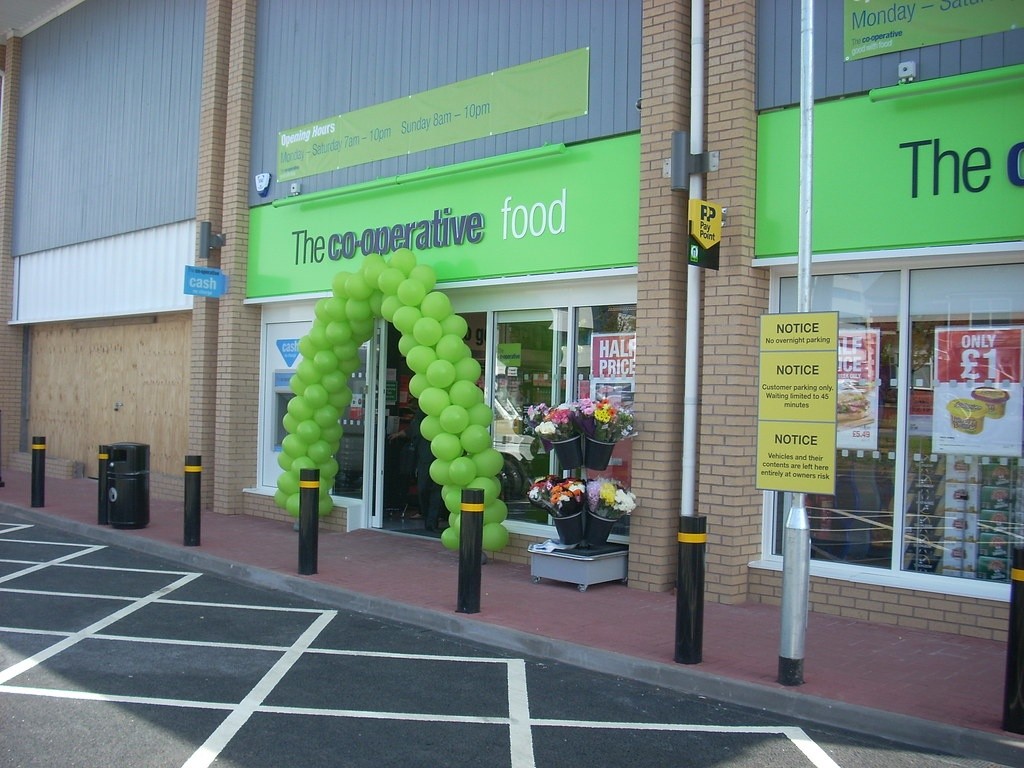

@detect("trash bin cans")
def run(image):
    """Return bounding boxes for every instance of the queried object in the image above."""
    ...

[107,442,151,529]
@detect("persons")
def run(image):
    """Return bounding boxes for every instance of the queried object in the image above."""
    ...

[386,404,442,532]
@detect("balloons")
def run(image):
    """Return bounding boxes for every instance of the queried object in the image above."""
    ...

[276,251,511,555]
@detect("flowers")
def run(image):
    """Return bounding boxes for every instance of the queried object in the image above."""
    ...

[586,476,637,519]
[524,401,639,443]
[526,475,586,517]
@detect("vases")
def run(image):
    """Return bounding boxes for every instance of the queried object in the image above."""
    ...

[550,436,583,470]
[584,437,616,471]
[553,511,583,545]
[584,511,619,547]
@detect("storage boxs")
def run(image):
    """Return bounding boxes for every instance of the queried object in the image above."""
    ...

[944,511,980,542]
[979,509,1015,534]
[981,465,1019,488]
[978,533,1014,557]
[943,540,978,571]
[945,482,981,513]
[946,454,982,484]
[977,556,1013,583]
[980,486,1017,511]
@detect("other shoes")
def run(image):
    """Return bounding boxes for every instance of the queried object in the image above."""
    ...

[426,526,444,534]
[410,514,425,520]
